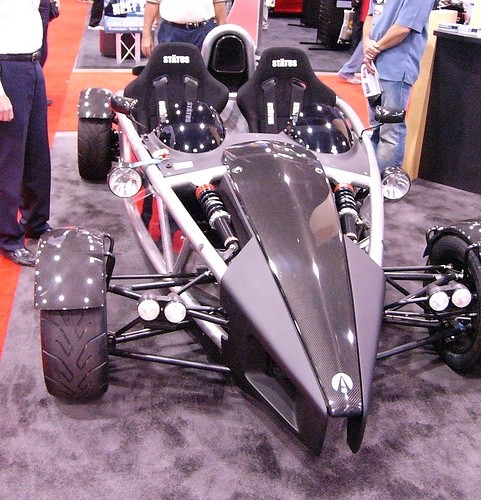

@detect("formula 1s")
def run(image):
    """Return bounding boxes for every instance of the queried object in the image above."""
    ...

[32,24,481,457]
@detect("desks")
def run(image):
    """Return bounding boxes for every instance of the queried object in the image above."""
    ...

[419,28,480,194]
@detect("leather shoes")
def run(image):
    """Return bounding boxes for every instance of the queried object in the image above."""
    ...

[0,246,37,267]
[26,228,55,240]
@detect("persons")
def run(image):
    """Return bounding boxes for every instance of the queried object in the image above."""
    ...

[261,0,276,30]
[0,0,58,267]
[38,0,52,107]
[353,0,435,200]
[337,1,475,84]
[140,1,228,59]
[88,0,105,31]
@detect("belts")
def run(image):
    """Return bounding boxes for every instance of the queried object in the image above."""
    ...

[0,48,43,62]
[162,17,214,31]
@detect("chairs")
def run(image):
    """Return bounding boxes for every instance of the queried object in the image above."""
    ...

[124,42,229,135]
[236,47,337,133]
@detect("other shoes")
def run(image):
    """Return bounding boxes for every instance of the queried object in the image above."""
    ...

[354,73,361,77]
[345,76,361,84]
